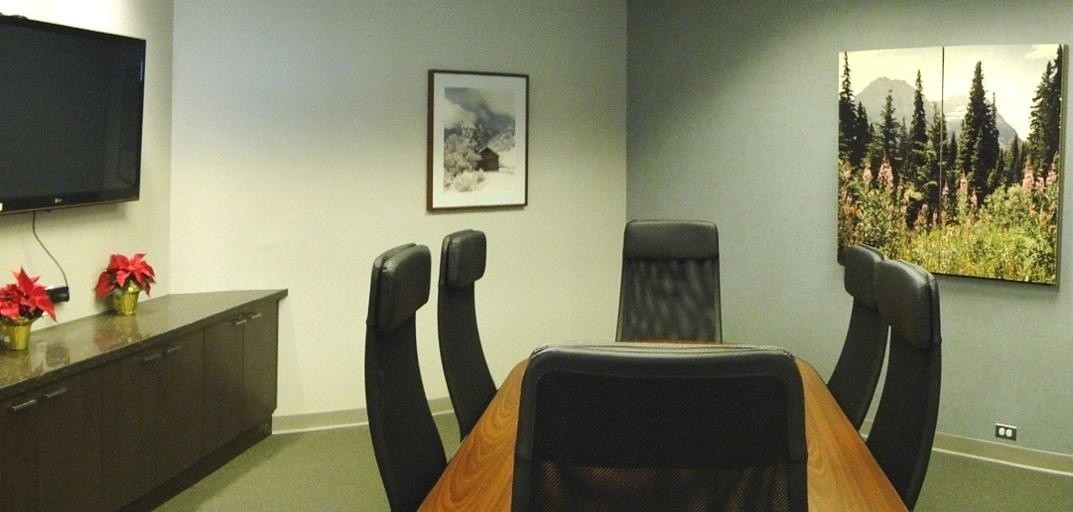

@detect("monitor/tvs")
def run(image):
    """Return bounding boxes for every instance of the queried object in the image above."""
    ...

[0,14,147,216]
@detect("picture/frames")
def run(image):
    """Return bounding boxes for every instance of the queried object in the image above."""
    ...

[427,68,530,214]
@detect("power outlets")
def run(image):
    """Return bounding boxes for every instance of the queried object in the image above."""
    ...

[994,423,1017,441]
[46,286,69,306]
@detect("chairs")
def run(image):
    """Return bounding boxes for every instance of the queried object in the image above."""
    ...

[826,244,889,432]
[364,242,447,512]
[612,219,724,344]
[865,258,944,512]
[508,341,807,512]
[437,227,499,443]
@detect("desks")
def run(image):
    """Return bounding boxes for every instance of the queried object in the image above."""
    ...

[416,354,911,512]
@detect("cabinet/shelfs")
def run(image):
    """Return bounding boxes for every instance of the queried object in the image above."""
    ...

[203,300,279,478]
[102,328,203,512]
[0,365,102,512]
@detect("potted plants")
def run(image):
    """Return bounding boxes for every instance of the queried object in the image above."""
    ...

[95,252,155,315]
[0,267,57,350]
